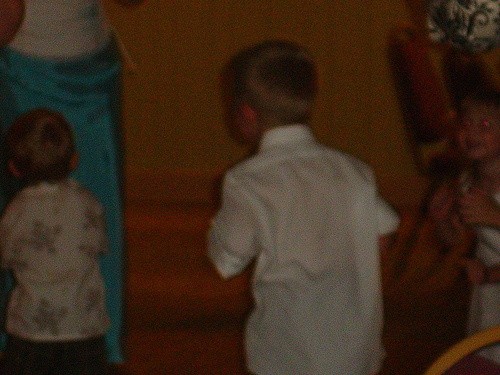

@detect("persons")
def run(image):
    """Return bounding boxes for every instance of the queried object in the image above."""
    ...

[204,35,397,374]
[2,108,113,373]
[0,0,136,369]
[419,81,500,375]
[391,1,500,190]
